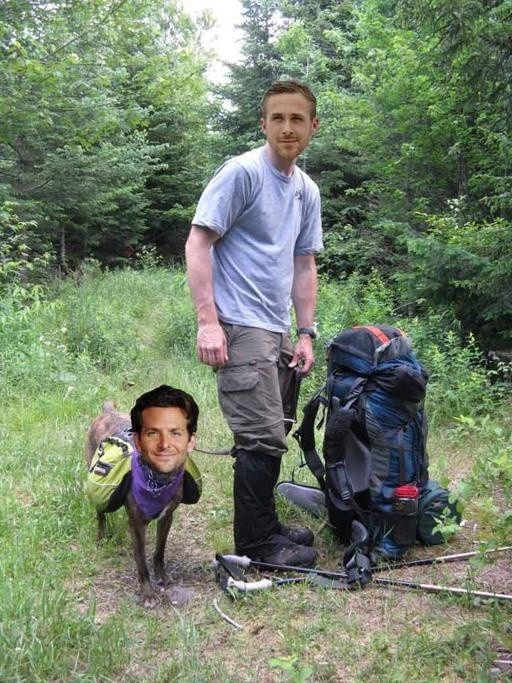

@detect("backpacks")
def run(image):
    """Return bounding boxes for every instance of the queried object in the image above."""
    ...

[293,322,464,585]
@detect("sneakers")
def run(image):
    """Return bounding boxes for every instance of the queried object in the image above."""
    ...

[243,524,318,571]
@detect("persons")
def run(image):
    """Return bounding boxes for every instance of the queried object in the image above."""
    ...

[183,82,328,571]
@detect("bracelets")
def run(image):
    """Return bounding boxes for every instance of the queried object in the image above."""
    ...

[296,327,317,340]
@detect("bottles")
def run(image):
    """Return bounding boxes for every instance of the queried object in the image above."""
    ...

[393,484,419,549]
